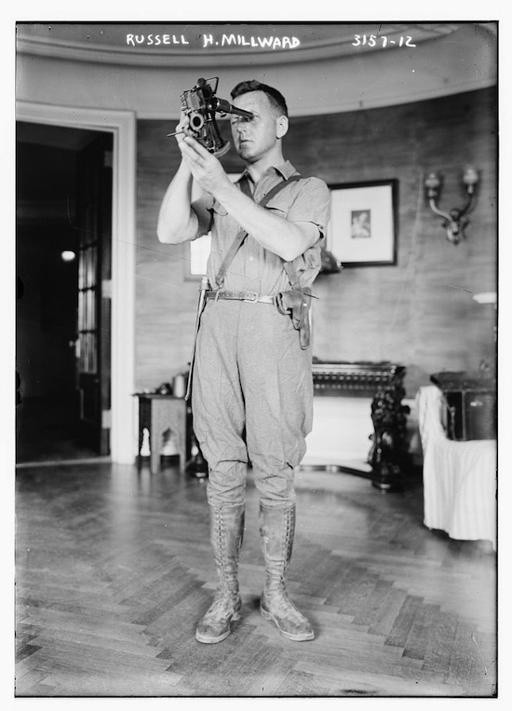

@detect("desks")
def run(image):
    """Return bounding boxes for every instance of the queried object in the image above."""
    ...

[136,392,187,475]
[186,360,411,492]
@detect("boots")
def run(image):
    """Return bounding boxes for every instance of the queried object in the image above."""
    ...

[196,502,244,643]
[257,501,314,641]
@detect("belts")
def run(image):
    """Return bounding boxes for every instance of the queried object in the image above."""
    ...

[206,287,275,306]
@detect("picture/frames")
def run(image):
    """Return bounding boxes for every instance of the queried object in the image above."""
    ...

[327,179,400,266]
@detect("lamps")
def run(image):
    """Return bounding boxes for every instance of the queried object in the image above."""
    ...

[426,169,479,244]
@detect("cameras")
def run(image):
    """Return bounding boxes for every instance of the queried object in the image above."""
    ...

[180,84,225,154]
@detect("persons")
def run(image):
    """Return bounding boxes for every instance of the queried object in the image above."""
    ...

[156,79,332,643]
